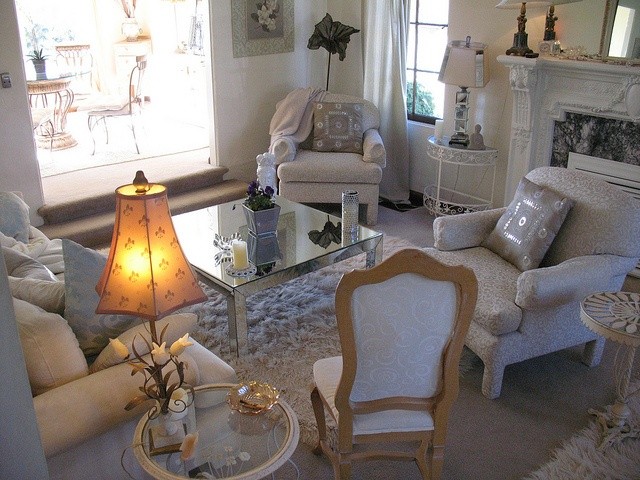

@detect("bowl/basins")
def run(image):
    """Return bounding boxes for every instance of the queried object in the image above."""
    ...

[225,378,281,415]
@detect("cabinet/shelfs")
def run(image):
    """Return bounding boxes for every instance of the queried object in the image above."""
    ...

[423,135,498,221]
[114,36,153,86]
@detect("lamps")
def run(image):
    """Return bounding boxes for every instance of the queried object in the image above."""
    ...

[436,34,490,148]
[95,170,209,454]
[496,0,551,56]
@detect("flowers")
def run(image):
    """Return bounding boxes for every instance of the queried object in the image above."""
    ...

[243,181,276,212]
[251,1,279,32]
[254,262,277,276]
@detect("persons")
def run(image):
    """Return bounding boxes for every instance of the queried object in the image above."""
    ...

[467,124,486,150]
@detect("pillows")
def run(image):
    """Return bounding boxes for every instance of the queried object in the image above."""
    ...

[90,311,197,373]
[9,275,66,314]
[63,238,143,349]
[1,247,61,282]
[1,189,33,243]
[312,100,362,156]
[481,175,577,276]
[10,299,90,392]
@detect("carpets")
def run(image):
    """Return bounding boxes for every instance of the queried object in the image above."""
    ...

[83,229,477,449]
[516,372,640,480]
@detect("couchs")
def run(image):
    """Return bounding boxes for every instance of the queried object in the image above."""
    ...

[0,187,235,480]
[416,168,640,402]
[273,95,386,224]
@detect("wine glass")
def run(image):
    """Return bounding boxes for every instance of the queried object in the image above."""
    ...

[341,190,359,236]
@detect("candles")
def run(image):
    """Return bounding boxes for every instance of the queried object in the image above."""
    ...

[232,240,248,271]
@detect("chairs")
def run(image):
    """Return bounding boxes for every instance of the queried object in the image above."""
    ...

[309,249,476,480]
[56,40,97,113]
[76,54,149,157]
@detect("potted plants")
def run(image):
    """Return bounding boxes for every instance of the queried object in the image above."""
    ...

[118,0,140,39]
[26,47,52,82]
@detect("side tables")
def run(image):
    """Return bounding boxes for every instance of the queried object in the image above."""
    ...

[132,383,301,480]
[577,290,640,454]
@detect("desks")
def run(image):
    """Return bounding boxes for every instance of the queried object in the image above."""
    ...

[25,77,78,149]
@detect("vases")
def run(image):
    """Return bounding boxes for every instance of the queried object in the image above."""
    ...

[246,231,282,265]
[241,201,280,236]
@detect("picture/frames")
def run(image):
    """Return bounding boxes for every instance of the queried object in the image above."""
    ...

[231,0,295,58]
